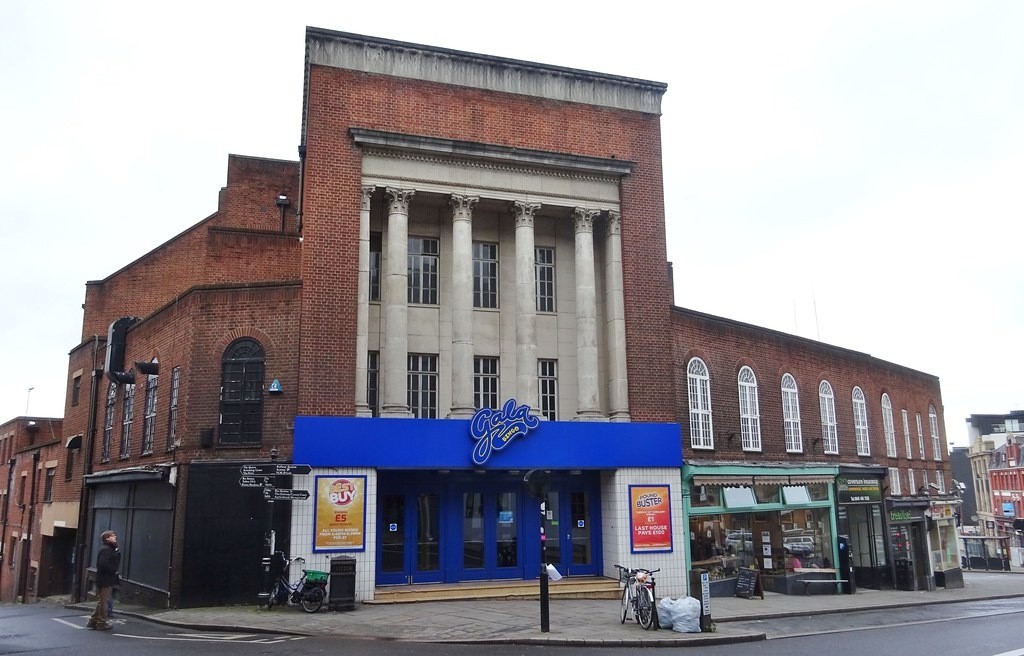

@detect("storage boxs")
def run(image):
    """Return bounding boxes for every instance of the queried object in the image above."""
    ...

[306,569,329,582]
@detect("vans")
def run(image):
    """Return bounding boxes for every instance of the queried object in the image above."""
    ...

[724,532,757,552]
[782,537,814,554]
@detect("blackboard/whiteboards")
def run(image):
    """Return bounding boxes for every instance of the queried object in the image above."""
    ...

[734,567,764,595]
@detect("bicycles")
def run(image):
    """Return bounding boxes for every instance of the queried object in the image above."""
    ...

[264,550,324,613]
[614,561,653,630]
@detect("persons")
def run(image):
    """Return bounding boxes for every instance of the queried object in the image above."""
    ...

[86,529,120,631]
[107,543,121,618]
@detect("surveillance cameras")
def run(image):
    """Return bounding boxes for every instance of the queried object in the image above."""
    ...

[959,482,965,490]
[930,482,940,490]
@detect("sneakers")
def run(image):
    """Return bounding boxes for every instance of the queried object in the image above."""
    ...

[87,620,113,630]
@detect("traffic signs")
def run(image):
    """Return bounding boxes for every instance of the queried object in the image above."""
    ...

[238,464,312,502]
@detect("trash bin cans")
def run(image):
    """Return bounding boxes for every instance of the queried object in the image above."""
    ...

[329,555,357,613]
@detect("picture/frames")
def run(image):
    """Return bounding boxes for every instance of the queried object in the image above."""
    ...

[312,475,367,554]
[628,484,674,554]
